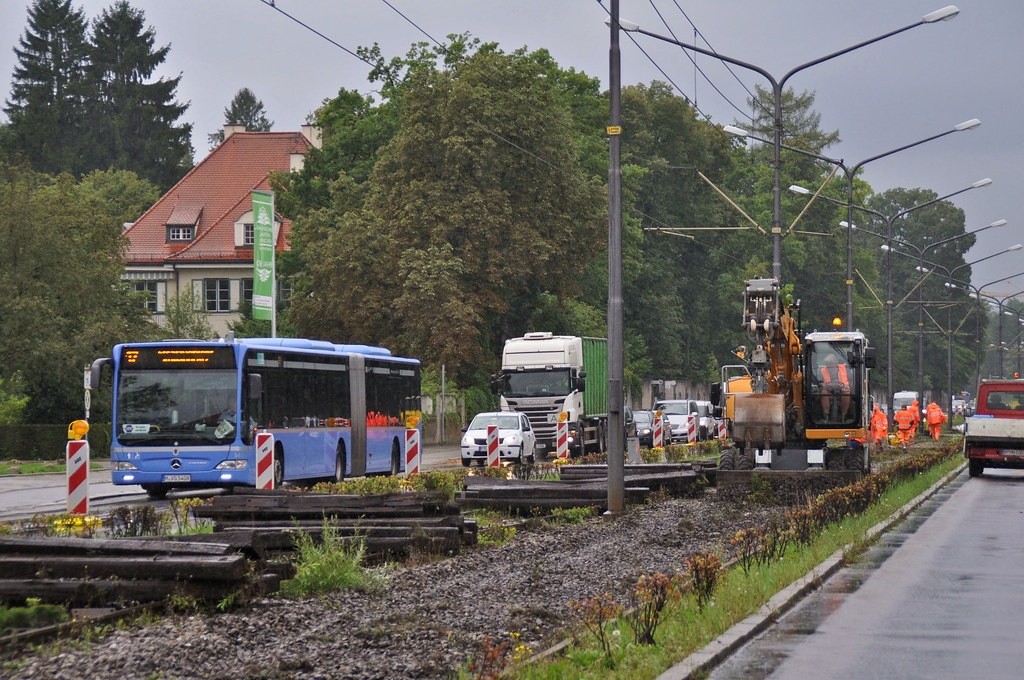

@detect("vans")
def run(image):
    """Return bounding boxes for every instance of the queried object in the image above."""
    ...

[652,398,700,443]
[893,392,919,414]
[694,400,714,441]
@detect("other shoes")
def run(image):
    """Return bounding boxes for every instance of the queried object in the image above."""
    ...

[841,416,846,421]
[903,446,907,453]
[880,447,883,452]
[824,417,828,422]
[876,449,880,452]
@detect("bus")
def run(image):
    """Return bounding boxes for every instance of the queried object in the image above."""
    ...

[89,331,422,499]
[710,275,878,493]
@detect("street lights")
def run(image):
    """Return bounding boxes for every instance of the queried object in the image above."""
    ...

[839,217,1008,427]
[716,117,981,329]
[917,266,1023,376]
[610,5,964,306]
[785,176,994,432]
[881,243,1023,428]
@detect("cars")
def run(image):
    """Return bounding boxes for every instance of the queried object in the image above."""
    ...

[460,411,537,466]
[951,400,966,413]
[623,406,637,451]
[632,411,658,448]
[654,411,672,444]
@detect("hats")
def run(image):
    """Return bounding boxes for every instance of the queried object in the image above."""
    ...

[227,394,236,400]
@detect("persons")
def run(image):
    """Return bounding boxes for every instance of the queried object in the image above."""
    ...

[908,401,920,444]
[990,394,1012,409]
[305,415,319,428]
[892,404,914,454]
[870,404,888,455]
[818,354,853,422]
[925,402,948,441]
[367,411,398,427]
[549,374,568,394]
[217,393,255,435]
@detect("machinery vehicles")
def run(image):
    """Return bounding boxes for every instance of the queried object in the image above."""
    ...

[963,372,1024,476]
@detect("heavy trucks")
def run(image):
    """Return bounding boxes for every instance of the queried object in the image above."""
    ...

[491,331,608,458]
